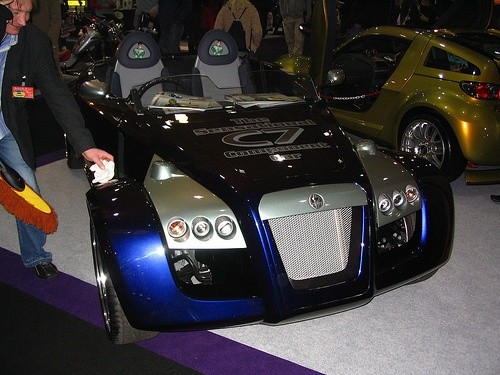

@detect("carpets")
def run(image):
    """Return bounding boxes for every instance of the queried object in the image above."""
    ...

[0,151,500,375]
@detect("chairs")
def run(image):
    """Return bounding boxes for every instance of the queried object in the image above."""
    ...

[111,32,178,109]
[191,30,255,99]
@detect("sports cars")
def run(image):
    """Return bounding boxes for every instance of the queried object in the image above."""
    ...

[270,0,500,181]
[62,27,457,344]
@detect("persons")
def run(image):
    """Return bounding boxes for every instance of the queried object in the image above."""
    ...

[132,0,500,57]
[0,0,115,280]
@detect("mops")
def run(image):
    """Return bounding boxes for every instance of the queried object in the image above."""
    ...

[0,157,60,233]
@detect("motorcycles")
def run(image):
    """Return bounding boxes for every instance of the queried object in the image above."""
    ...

[57,10,160,71]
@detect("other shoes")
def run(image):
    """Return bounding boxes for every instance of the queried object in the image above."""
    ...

[35,261,60,282]
[491,194,500,204]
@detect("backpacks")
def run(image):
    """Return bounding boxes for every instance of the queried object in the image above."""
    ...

[226,5,249,51]
